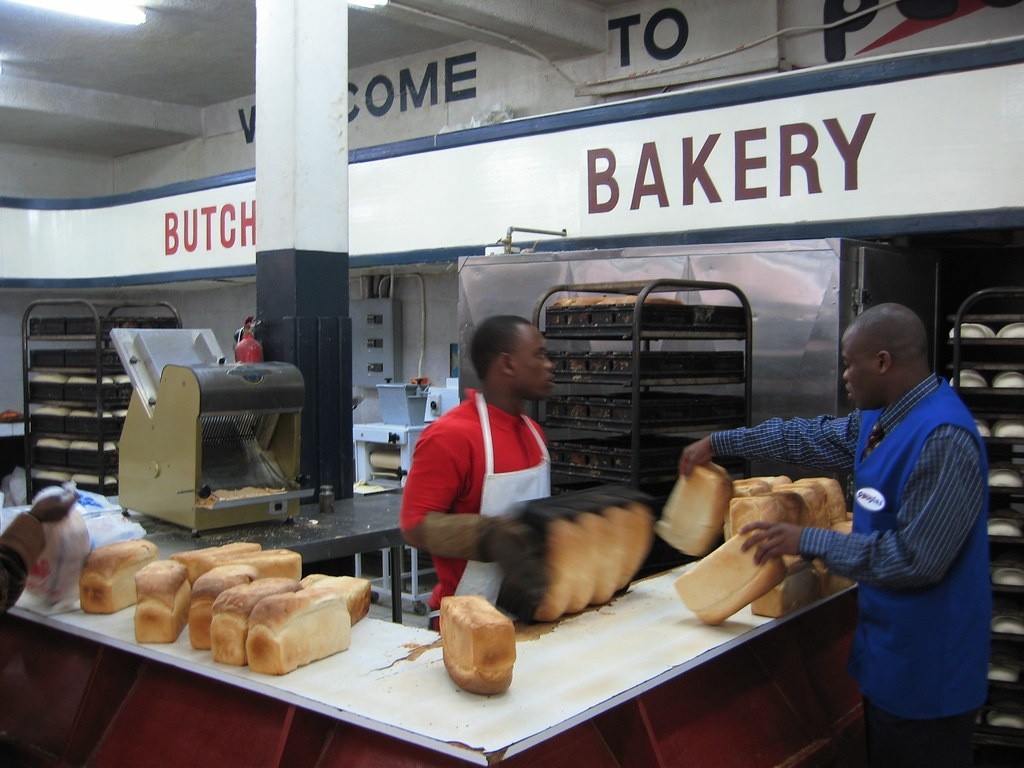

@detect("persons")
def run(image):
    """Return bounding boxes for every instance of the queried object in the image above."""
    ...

[680,304,991,767]
[399,315,557,633]
[0,487,77,617]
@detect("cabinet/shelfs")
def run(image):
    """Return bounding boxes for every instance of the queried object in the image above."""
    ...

[530,278,753,562]
[946,287,1024,749]
[21,299,183,506]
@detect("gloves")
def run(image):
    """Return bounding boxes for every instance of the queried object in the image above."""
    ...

[425,512,540,575]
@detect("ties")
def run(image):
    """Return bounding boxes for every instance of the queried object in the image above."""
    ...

[860,422,884,462]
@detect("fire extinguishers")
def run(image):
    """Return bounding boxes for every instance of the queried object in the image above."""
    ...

[232,313,264,364]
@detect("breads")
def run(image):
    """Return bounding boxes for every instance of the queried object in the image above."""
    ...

[653,459,858,626]
[439,595,517,695]
[34,373,131,486]
[551,296,685,306]
[945,322,1024,729]
[533,501,652,622]
[135,542,372,675]
[21,508,91,605]
[78,538,159,615]
